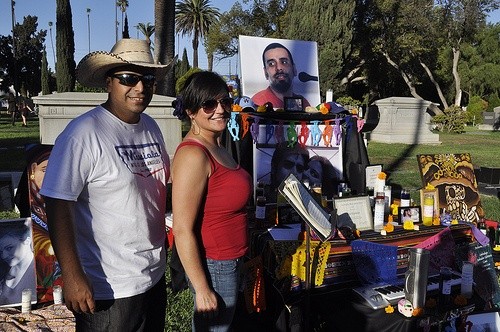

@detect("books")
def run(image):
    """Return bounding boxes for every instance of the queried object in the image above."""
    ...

[283,174,332,238]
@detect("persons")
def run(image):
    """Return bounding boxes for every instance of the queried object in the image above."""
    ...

[251,43,311,110]
[40,38,171,332]
[6,92,32,127]
[264,141,338,203]
[171,71,254,332]
[402,209,411,222]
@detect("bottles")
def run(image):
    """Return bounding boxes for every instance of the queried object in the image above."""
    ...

[21,288,32,312]
[383,186,391,222]
[462,264,473,285]
[53,285,63,304]
[401,189,410,207]
[423,196,433,226]
[256,196,266,228]
[373,192,385,232]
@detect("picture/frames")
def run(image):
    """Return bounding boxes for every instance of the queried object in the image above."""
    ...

[398,206,421,224]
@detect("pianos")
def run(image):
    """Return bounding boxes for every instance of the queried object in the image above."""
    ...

[349,269,477,310]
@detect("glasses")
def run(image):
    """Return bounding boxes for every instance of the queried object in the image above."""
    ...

[108,74,156,90]
[197,97,232,113]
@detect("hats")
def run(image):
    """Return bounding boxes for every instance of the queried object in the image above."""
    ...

[75,39,174,88]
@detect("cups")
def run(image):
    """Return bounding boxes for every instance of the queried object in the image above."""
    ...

[405,248,430,308]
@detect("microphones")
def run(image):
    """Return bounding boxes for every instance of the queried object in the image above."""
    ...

[298,72,318,83]
